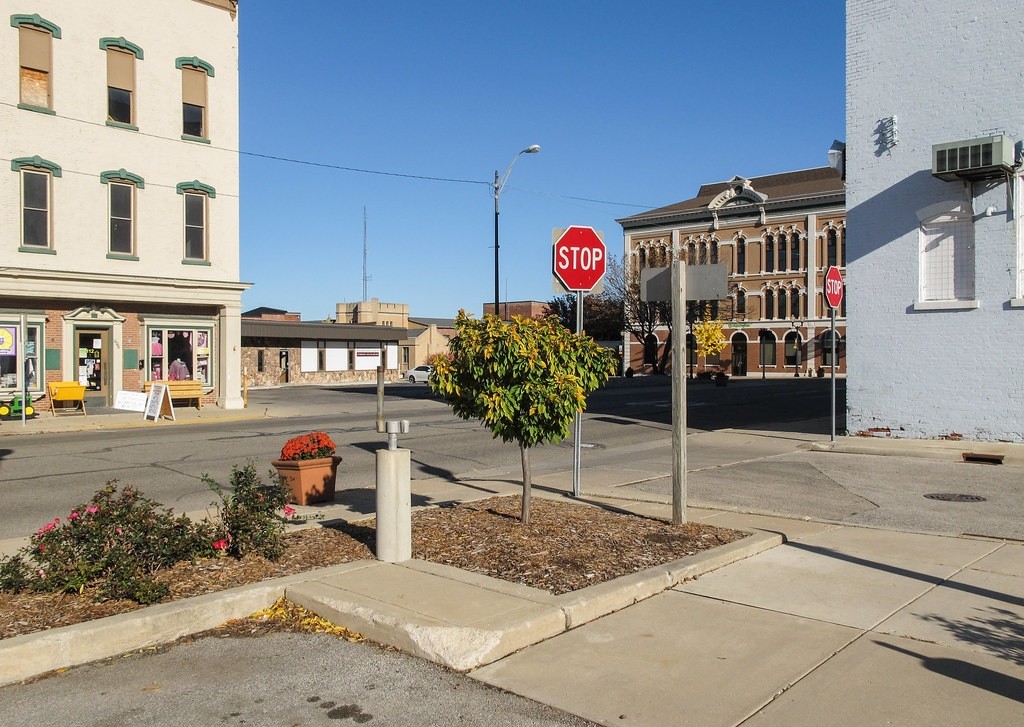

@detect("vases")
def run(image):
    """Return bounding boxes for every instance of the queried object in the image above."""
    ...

[272,455,343,505]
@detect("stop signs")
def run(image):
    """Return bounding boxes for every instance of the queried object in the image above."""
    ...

[824,266,844,309]
[554,226,606,292]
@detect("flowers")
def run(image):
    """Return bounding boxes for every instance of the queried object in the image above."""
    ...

[279,431,337,460]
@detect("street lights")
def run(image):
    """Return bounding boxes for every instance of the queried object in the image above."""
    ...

[790,314,804,377]
[494,144,541,318]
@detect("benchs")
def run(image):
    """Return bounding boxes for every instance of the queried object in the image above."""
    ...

[145,380,203,411]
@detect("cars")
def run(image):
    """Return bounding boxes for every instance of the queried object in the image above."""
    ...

[405,365,439,384]
